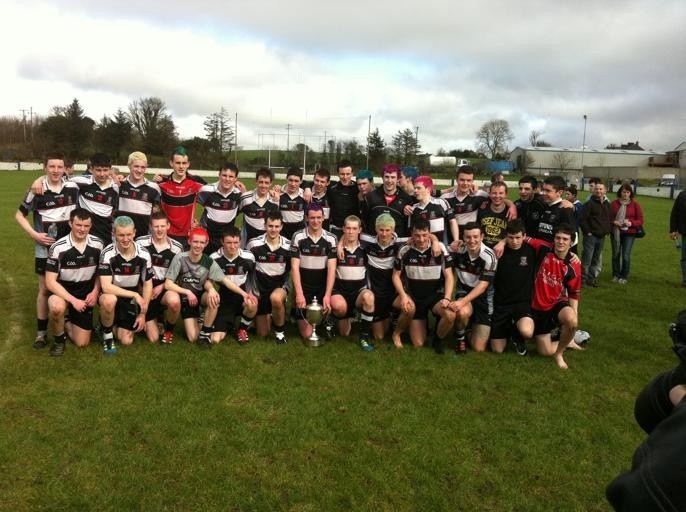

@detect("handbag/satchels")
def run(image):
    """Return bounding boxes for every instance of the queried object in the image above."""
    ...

[634,226,645,237]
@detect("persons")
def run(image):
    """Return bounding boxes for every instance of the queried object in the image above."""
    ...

[606,364,685,506]
[16,147,583,369]
[670,189,685,287]
[565,176,643,287]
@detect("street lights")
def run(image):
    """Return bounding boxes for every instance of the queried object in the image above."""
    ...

[581,115,588,176]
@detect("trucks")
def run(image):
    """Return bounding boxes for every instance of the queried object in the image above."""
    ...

[424,155,469,170]
[662,174,677,188]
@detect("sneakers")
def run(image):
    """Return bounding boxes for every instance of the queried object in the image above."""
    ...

[610,276,618,282]
[273,331,288,344]
[515,339,528,356]
[618,278,627,284]
[359,336,375,352]
[198,337,212,346]
[50,341,66,356]
[236,328,249,345]
[324,319,337,341]
[454,334,466,353]
[33,330,48,349]
[588,278,600,286]
[198,310,204,323]
[91,321,103,339]
[390,318,400,329]
[102,338,118,353]
[161,328,173,343]
[158,322,165,335]
